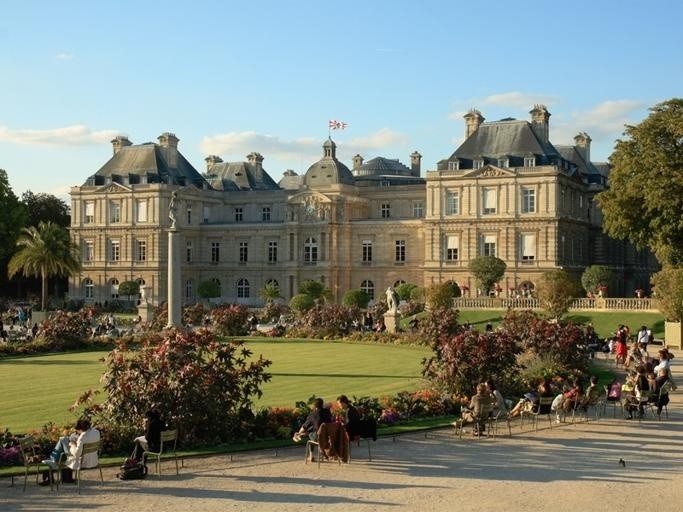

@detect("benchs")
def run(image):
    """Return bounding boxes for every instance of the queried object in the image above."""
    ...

[184,435,304,468]
[380,412,454,443]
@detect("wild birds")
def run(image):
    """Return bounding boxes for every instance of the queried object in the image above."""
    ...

[619,456,626,467]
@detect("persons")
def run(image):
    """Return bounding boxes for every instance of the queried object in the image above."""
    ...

[41,419,101,470]
[1,303,167,349]
[449,322,674,436]
[337,310,386,337]
[185,311,292,334]
[292,397,332,461]
[129,410,163,462]
[408,315,493,336]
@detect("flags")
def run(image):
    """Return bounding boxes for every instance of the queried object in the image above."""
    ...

[328,120,350,130]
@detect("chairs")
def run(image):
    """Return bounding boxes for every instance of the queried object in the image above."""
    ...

[347,418,377,461]
[55,437,107,494]
[141,428,180,479]
[19,433,53,488]
[306,420,344,468]
[454,333,675,444]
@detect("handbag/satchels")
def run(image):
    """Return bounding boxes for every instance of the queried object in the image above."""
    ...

[607,378,620,400]
[119,464,148,481]
